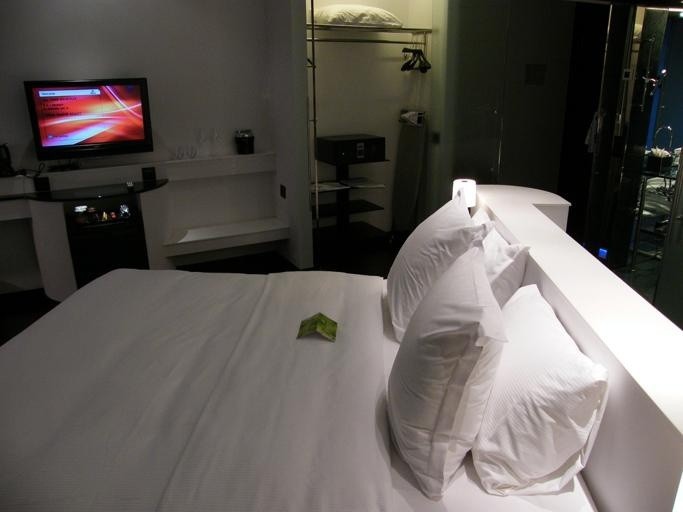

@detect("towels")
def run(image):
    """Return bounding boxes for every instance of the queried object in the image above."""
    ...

[312,2,405,25]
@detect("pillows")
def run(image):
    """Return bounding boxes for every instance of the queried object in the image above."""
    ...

[384,197,484,345]
[471,282,607,500]
[386,247,509,500]
[481,227,531,308]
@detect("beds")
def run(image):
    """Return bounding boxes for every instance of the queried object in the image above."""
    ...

[1,197,683,507]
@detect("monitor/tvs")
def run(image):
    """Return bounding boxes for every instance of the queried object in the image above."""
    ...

[22,76,153,162]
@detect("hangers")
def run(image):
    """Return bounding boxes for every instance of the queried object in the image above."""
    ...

[401,33,430,72]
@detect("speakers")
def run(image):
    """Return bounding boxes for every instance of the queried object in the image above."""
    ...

[33,175,51,194]
[141,167,156,187]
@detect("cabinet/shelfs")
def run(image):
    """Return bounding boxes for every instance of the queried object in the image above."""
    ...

[0,151,314,301]
